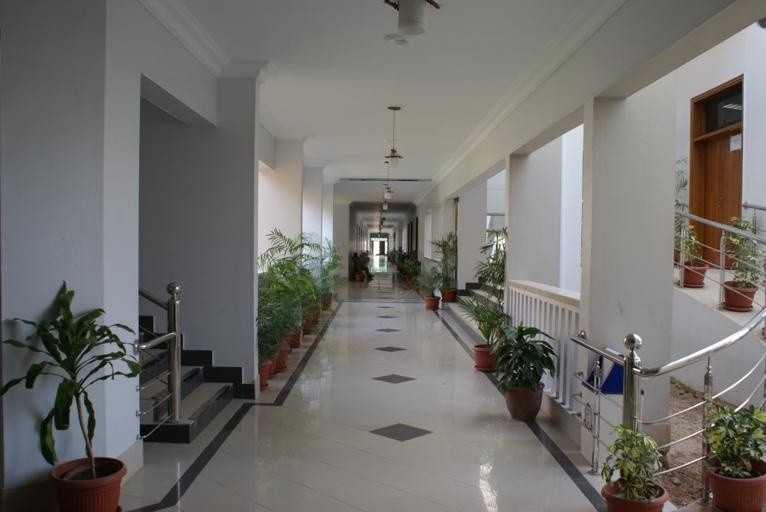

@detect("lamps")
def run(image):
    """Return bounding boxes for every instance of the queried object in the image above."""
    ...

[382,106,403,210]
[385,0,441,35]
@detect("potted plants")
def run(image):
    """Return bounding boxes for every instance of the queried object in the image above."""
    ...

[429,232,457,302]
[411,267,442,310]
[256,228,344,391]
[0,280,159,512]
[671,164,689,264]
[722,215,759,311]
[703,402,766,512]
[352,250,370,282]
[389,247,421,290]
[459,224,508,371]
[599,423,669,512]
[679,225,708,288]
[491,323,556,420]
